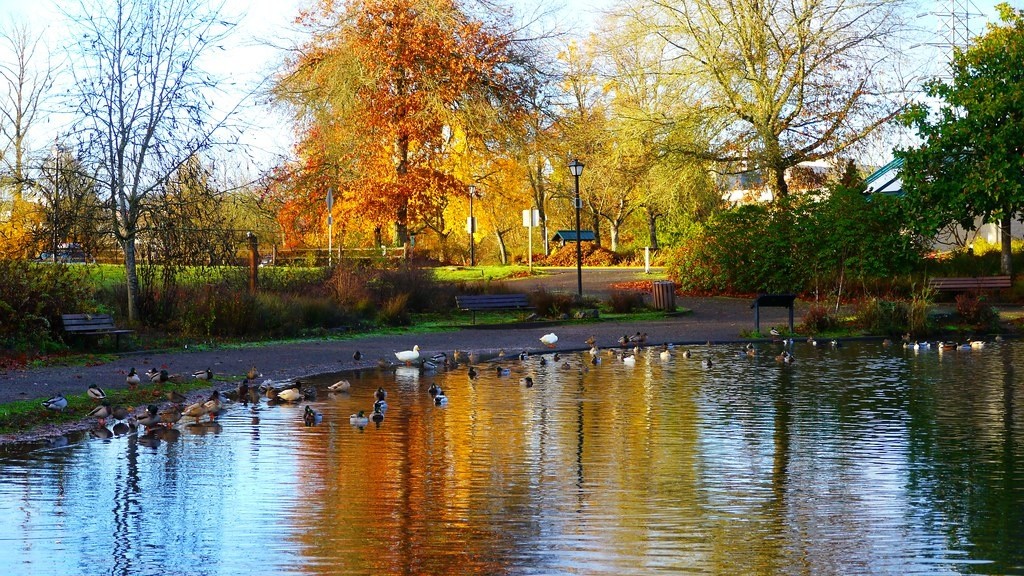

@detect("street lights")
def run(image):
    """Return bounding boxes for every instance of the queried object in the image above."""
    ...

[568,158,585,294]
[49,143,66,263]
[468,184,478,267]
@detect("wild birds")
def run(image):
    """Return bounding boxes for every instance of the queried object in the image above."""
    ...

[38,326,989,447]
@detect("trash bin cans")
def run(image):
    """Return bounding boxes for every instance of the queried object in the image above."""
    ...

[654,280,675,311]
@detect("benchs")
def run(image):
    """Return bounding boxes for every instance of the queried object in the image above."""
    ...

[62,313,134,352]
[455,293,537,324]
[926,275,1011,304]
